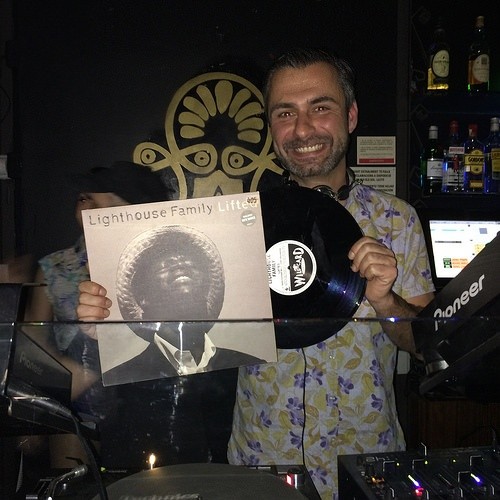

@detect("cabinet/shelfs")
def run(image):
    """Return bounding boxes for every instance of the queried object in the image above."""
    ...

[395,0,500,220]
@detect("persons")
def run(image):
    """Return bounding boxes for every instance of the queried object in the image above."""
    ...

[78,45,436,500]
[13,165,170,473]
[102,249,269,389]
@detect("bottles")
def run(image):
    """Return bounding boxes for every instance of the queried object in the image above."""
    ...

[420,125,444,195]
[424,28,450,96]
[441,150,460,192]
[447,120,464,153]
[463,124,485,192]
[467,16,490,95]
[484,117,500,193]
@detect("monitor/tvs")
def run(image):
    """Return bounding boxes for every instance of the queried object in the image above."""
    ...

[428,219,500,279]
